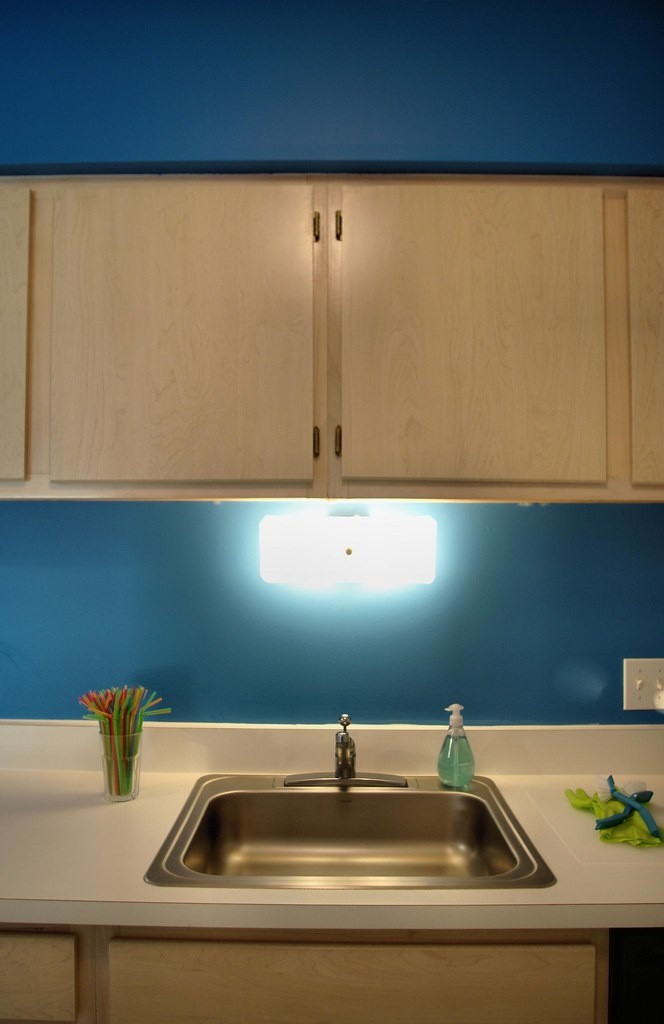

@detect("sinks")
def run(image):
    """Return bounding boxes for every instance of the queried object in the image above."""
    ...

[143,772,561,892]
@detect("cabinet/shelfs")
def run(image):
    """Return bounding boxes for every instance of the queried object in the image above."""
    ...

[1,174,663,504]
[0,923,610,1024]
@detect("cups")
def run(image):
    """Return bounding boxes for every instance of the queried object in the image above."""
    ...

[100,730,144,801]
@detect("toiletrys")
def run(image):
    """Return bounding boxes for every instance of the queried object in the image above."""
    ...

[437,703,475,787]
[333,714,358,793]
